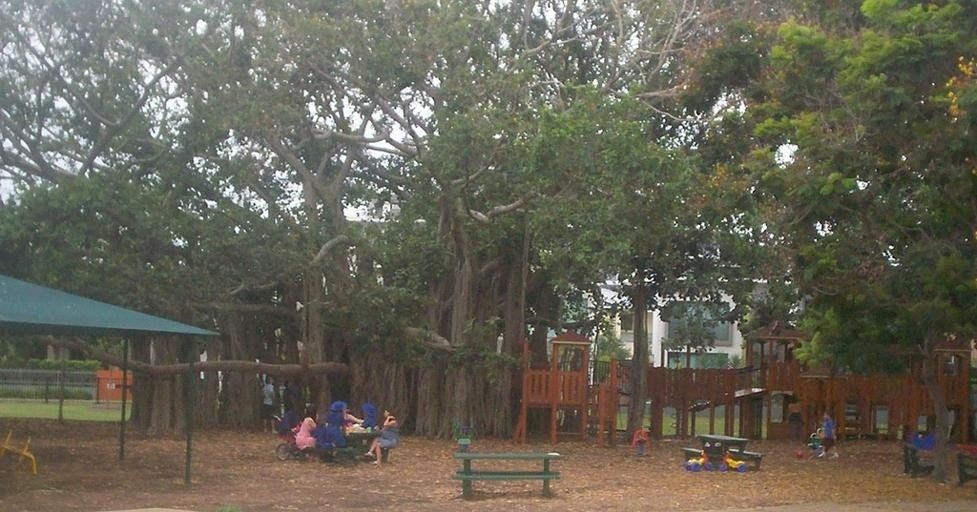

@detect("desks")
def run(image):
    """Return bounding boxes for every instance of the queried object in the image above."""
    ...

[696,434,752,469]
[345,427,389,464]
[453,451,559,496]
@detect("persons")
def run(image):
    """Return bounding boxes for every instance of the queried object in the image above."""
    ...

[275,401,401,466]
[262,375,278,435]
[817,408,840,459]
[634,426,651,456]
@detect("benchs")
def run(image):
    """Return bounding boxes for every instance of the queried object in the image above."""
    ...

[451,470,560,499]
[679,447,704,461]
[956,451,976,486]
[303,444,393,462]
[902,442,934,478]
[728,448,765,470]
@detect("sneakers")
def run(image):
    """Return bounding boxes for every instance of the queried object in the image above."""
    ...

[369,461,377,465]
[818,452,827,458]
[365,453,373,458]
[829,452,839,460]
